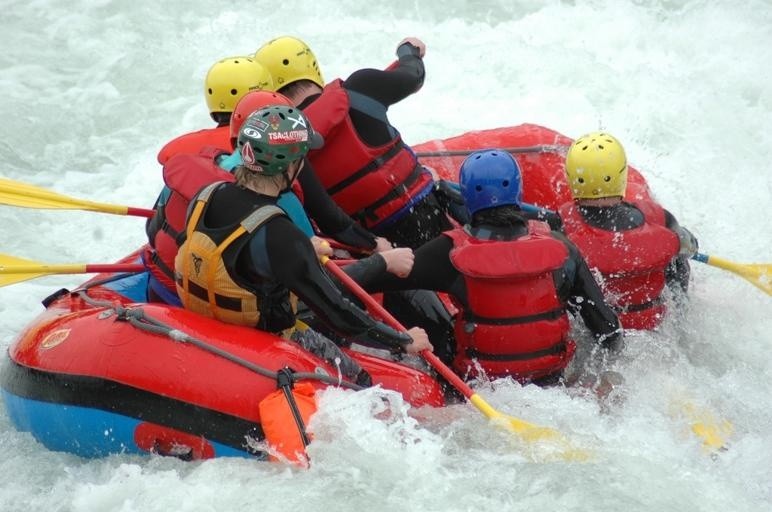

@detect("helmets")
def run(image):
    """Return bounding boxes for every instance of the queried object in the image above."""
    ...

[458,149,523,219]
[205,36,324,176]
[566,133,627,201]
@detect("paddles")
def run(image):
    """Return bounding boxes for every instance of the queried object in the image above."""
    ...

[0,255,366,286]
[443,179,768,292]
[0,180,374,253]
[322,257,536,432]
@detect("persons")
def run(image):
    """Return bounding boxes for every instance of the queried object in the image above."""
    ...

[254,33,471,253]
[172,105,435,394]
[145,58,396,261]
[349,150,620,399]
[147,90,333,309]
[520,131,680,336]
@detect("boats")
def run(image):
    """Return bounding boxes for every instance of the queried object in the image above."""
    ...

[0,122,680,460]
